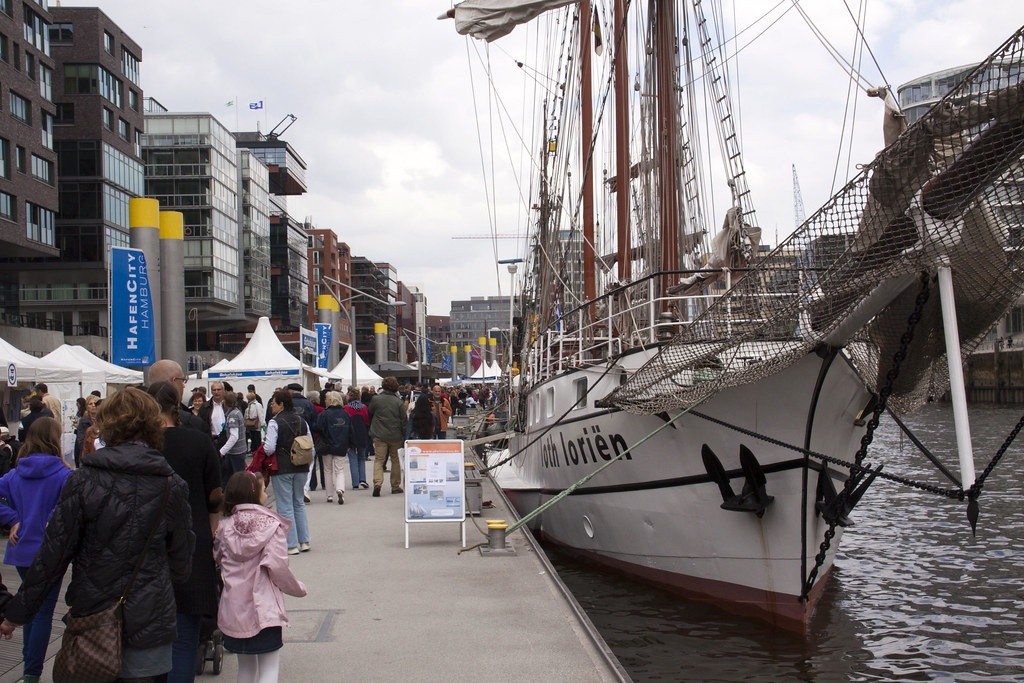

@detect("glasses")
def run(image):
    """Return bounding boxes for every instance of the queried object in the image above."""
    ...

[175,375,189,383]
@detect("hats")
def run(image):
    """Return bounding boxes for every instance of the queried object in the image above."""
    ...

[288,383,304,391]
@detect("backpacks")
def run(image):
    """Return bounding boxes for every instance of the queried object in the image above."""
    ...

[279,415,313,466]
[348,413,367,448]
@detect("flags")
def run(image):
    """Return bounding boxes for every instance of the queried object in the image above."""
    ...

[225,100,234,109]
[249,100,263,110]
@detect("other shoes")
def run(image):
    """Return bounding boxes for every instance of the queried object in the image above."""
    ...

[373,485,381,496]
[327,497,333,502]
[353,486,359,489]
[304,495,311,502]
[289,547,299,554]
[311,486,316,490]
[391,488,403,494]
[337,489,344,504]
[361,481,369,488]
[300,542,311,551]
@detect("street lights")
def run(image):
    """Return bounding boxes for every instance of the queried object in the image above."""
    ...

[401,327,450,383]
[321,275,408,388]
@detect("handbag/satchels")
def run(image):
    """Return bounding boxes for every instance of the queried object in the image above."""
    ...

[244,418,259,429]
[51,598,124,683]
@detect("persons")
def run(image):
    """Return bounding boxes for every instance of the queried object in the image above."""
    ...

[0,359,498,683]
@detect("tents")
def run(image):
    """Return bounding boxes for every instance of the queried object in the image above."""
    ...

[180,357,230,410]
[0,337,144,472]
[207,316,319,443]
[329,344,384,395]
[471,360,503,385]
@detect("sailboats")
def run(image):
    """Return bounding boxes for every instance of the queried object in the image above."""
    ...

[430,0,1024,644]
